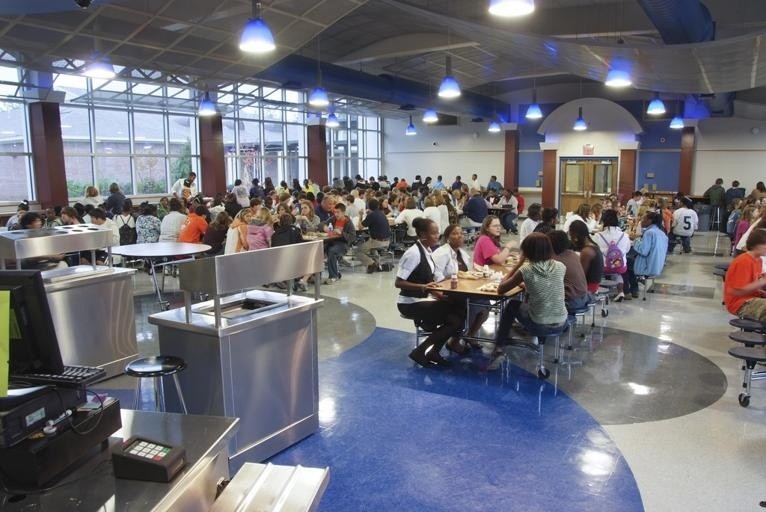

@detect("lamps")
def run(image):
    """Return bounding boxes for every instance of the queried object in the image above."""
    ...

[71,0,688,137]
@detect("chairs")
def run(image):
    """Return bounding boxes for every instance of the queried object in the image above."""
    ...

[46,186,766,417]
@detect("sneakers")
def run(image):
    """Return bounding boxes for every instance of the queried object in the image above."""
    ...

[425,350,449,365]
[408,349,434,368]
[486,347,505,372]
[307,271,344,285]
[297,278,309,292]
[366,260,383,274]
[164,265,171,275]
[624,289,640,300]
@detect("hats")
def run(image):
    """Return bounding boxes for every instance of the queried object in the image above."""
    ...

[680,196,693,206]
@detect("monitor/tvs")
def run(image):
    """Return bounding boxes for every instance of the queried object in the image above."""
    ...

[0,264,63,379]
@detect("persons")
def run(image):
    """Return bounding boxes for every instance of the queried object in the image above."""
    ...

[394,188,700,369]
[8,172,525,291]
[704,178,766,322]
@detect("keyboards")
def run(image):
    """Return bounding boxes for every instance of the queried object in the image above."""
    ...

[14,367,108,391]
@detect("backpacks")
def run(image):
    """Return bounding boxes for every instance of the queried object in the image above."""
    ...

[598,232,624,274]
[119,215,134,246]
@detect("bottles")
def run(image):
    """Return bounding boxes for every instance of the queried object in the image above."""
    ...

[483,265,490,279]
[450,272,458,288]
[327,223,333,231]
[296,223,300,228]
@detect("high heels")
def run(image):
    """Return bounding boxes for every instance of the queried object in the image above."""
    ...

[445,342,471,356]
[612,292,625,303]
[462,334,484,349]
